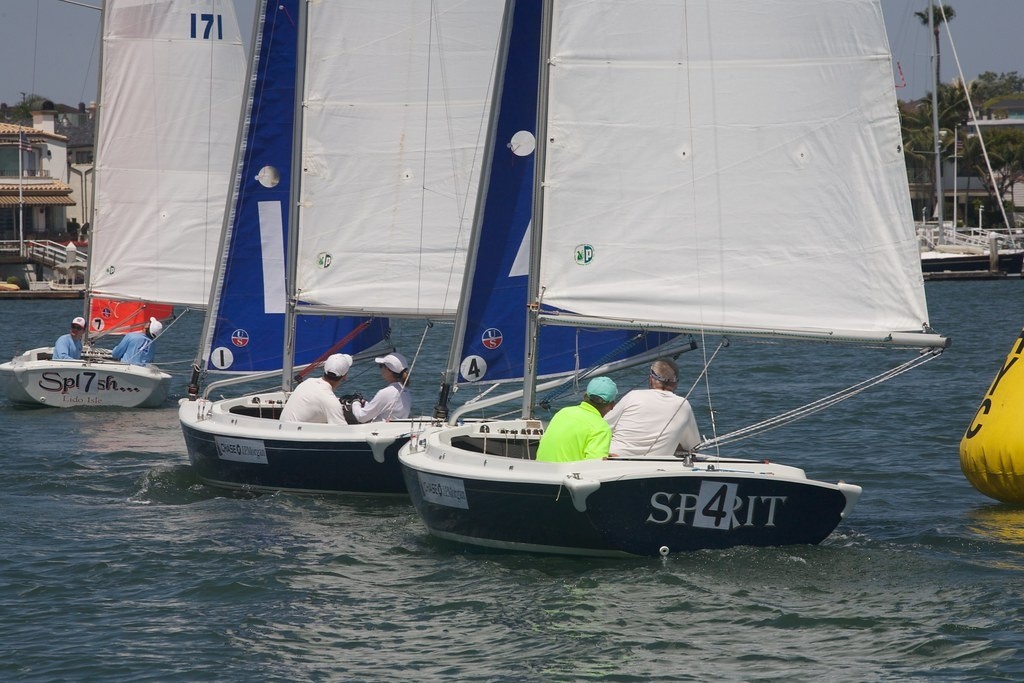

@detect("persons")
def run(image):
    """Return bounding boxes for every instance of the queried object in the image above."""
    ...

[112,316,162,364]
[280,353,353,425]
[51,317,86,360]
[535,376,617,462]
[604,358,702,459]
[350,352,412,423]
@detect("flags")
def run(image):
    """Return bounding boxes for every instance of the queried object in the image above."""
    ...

[19,132,32,151]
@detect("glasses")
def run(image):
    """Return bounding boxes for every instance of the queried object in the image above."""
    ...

[72,326,84,331]
[379,364,385,368]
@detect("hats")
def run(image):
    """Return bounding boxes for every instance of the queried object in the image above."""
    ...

[375,353,408,373]
[150,317,162,338]
[324,353,353,377]
[72,317,85,328]
[587,376,618,406]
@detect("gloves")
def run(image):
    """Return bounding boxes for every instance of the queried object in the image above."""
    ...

[350,397,366,407]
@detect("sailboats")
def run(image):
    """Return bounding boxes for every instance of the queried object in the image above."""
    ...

[897,0,1024,278]
[392,0,952,561]
[0,0,262,409]
[178,0,702,496]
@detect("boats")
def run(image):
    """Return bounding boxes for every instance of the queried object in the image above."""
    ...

[0,261,87,298]
